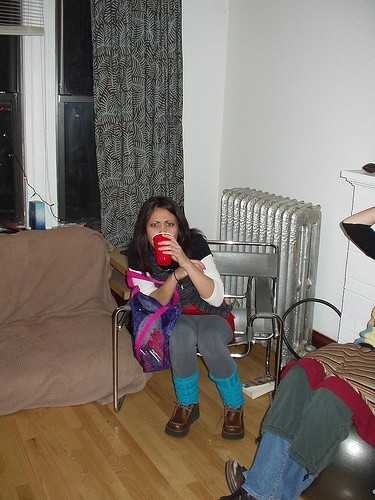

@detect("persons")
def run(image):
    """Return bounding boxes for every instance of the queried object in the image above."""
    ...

[128,195,245,439]
[220,206,375,500]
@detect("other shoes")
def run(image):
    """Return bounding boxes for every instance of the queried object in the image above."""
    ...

[218,459,258,500]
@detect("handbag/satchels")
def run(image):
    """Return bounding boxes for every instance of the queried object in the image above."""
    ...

[126,270,182,373]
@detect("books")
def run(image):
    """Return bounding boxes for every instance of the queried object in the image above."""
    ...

[241,374,276,399]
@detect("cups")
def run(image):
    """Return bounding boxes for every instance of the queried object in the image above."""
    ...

[151,232,173,266]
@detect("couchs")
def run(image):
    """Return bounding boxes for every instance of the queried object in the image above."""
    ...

[0,225,152,415]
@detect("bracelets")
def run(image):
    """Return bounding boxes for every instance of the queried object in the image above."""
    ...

[173,270,180,282]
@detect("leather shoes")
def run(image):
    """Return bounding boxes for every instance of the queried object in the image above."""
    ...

[221,405,244,439]
[166,400,200,437]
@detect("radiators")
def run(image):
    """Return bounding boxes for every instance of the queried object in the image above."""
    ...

[219,188,322,354]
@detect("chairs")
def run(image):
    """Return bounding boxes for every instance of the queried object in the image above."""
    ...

[112,240,284,413]
